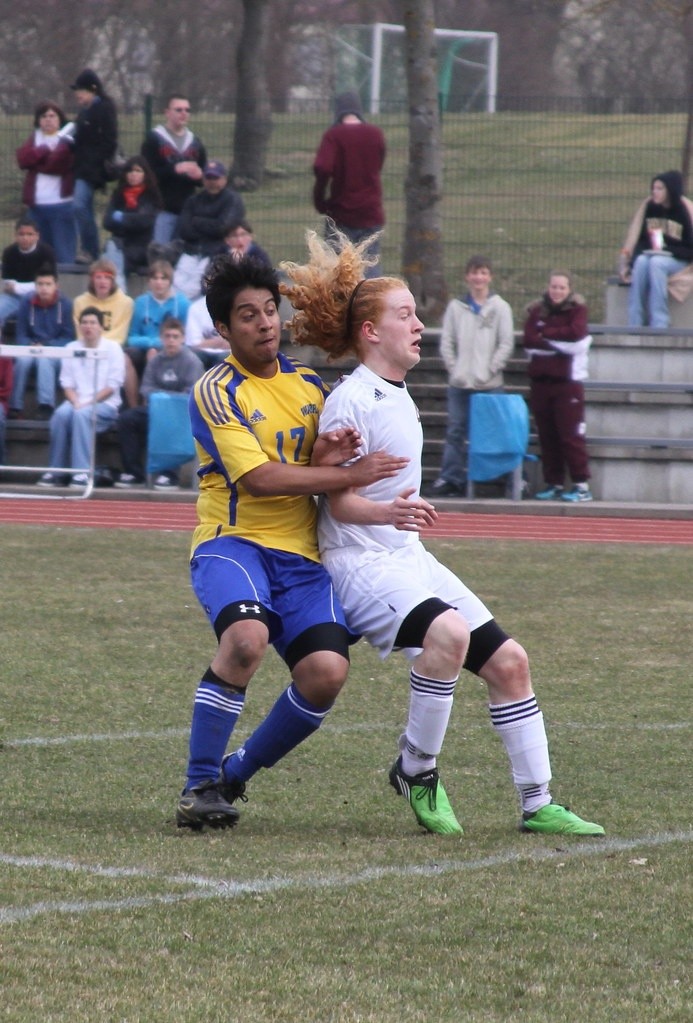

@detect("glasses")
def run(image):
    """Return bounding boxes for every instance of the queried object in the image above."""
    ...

[170,107,191,114]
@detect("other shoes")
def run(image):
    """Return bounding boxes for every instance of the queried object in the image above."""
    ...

[5,407,24,419]
[33,404,55,419]
[75,249,96,263]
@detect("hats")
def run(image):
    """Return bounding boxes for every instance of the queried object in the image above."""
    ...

[203,159,227,179]
[330,89,364,124]
[70,68,99,90]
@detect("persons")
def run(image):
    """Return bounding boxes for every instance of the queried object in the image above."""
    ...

[0,218,57,342]
[71,69,117,260]
[9,267,74,421]
[184,218,272,343]
[73,258,136,345]
[0,354,14,464]
[100,154,157,295]
[35,308,126,486]
[172,159,246,259]
[618,170,693,331]
[184,293,233,354]
[312,91,387,280]
[176,247,411,832]
[14,98,78,272]
[525,269,594,502]
[277,216,607,835]
[142,93,209,264]
[421,255,529,498]
[117,258,188,410]
[112,320,206,490]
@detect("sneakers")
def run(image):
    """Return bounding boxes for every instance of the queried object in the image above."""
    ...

[521,800,606,837]
[67,472,91,487]
[560,484,594,502]
[534,486,565,500]
[112,472,147,489]
[216,750,249,805]
[34,472,66,487]
[385,755,465,839]
[176,776,241,830]
[153,472,180,490]
[421,477,460,497]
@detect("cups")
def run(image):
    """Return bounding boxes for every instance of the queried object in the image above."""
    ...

[650,226,664,251]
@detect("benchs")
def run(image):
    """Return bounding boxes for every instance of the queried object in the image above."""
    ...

[0,256,232,484]
[572,272,693,499]
